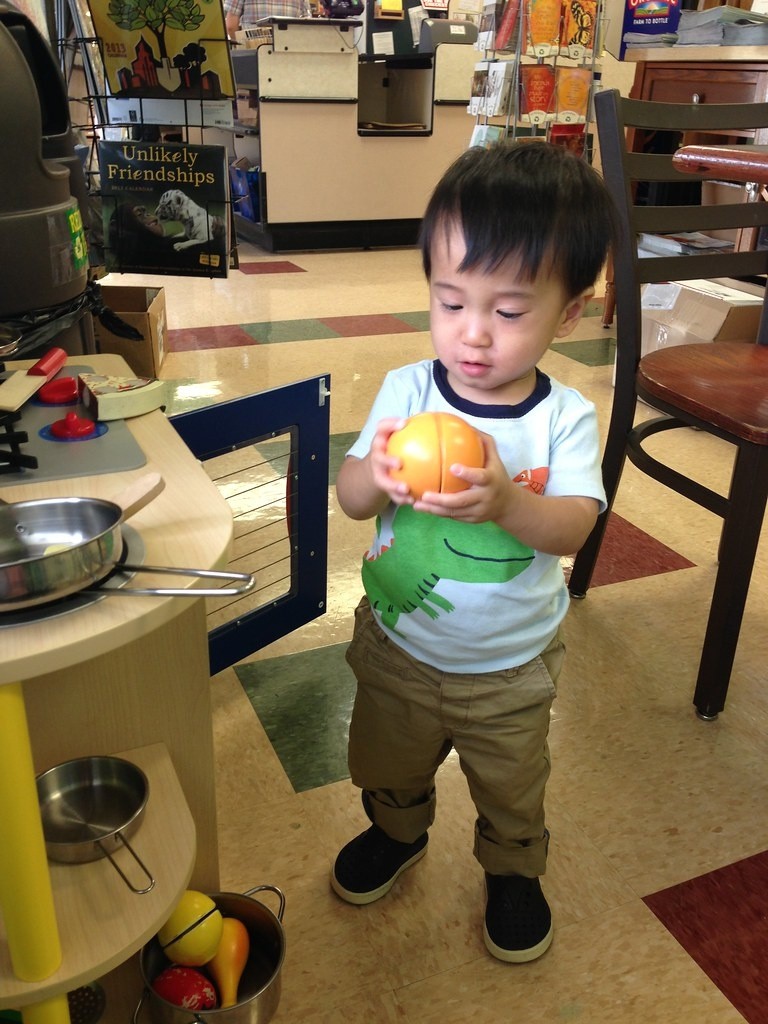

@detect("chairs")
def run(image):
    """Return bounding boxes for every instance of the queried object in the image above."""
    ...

[569,89,768,724]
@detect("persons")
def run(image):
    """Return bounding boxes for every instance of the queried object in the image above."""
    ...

[224,0,305,41]
[329,139,622,965]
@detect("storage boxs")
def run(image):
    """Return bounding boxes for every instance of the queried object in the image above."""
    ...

[57,286,168,380]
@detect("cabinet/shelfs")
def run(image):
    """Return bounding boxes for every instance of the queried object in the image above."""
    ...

[0,351,235,1024]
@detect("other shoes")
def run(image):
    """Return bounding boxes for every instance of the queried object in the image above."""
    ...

[484,870,553,963]
[332,824,429,905]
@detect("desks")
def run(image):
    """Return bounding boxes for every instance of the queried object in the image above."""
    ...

[624,45,768,204]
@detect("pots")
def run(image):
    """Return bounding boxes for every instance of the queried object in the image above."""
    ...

[35,756,155,895]
[134,885,286,1024]
[0,490,257,604]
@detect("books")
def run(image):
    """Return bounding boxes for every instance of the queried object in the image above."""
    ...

[102,66,236,129]
[94,138,241,279]
[642,232,736,252]
[85,0,239,101]
[673,278,764,306]
